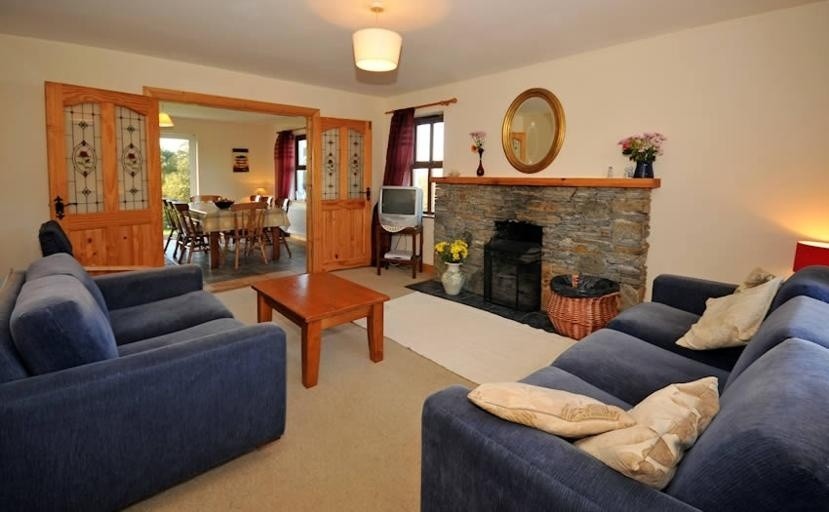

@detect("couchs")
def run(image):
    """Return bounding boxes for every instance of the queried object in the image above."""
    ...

[1,252,288,511]
[419,273,829,512]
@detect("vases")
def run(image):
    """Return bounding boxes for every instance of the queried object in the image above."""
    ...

[476,151,484,177]
[441,262,465,295]
[633,160,655,178]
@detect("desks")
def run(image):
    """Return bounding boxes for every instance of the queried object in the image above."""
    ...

[377,225,423,279]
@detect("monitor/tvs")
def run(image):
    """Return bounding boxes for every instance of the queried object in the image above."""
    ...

[378,185,423,228]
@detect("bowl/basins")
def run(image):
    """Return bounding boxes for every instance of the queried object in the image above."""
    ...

[215,201,233,209]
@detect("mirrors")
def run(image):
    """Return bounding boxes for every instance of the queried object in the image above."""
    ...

[500,88,567,174]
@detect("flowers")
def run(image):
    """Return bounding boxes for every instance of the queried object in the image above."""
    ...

[470,131,487,153]
[618,128,666,163]
[434,240,469,263]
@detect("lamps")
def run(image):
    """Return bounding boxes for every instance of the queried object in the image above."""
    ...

[792,241,828,274]
[352,7,404,73]
[159,101,175,128]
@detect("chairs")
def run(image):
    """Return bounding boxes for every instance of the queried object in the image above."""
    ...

[161,193,292,270]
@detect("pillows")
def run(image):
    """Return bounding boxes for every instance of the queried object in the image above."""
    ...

[674,276,786,350]
[467,376,720,491]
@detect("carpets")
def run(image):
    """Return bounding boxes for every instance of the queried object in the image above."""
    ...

[351,290,581,385]
